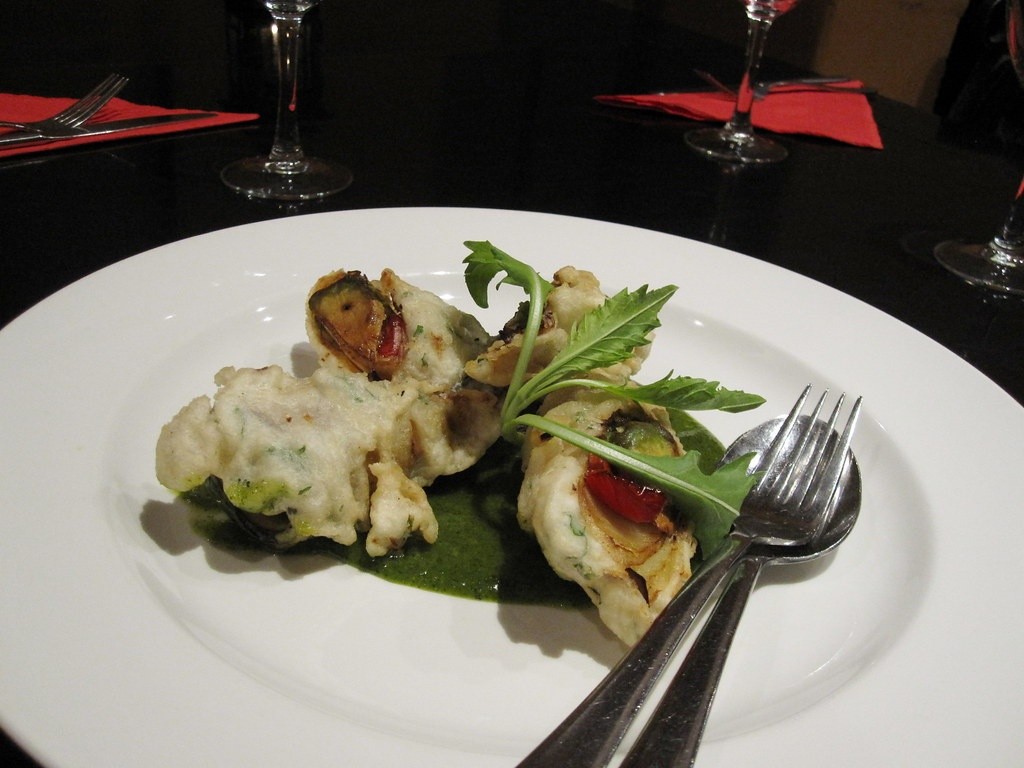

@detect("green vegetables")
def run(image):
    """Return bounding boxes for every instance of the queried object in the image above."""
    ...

[459,236,768,561]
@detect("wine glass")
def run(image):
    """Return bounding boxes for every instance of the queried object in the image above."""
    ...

[683,0,800,163]
[933,0,1024,295]
[218,1,354,202]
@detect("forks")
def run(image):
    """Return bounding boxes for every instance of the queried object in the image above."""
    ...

[518,384,864,768]
[0,73,129,132]
[694,69,878,100]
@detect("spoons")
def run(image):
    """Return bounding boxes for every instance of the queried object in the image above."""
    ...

[620,413,862,768]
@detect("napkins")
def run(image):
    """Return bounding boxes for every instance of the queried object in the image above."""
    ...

[592,79,884,150]
[0,93,260,158]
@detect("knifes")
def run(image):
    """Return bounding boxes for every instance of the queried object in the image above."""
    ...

[0,113,218,151]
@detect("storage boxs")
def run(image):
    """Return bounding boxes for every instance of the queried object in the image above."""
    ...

[664,0,969,114]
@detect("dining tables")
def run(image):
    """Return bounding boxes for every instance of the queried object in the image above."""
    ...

[0,0,1024,404]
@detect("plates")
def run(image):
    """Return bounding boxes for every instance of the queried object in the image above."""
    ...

[2,207,1024,765]
